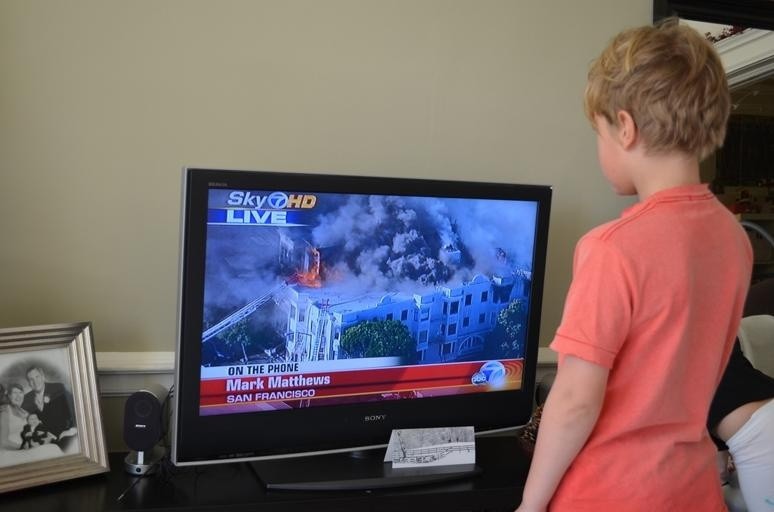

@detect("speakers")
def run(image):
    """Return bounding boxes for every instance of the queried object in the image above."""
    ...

[122,385,168,478]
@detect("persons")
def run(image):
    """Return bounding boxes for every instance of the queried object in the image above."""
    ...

[516,19,755,512]
[700,153,774,512]
[21,366,78,451]
[0,384,63,468]
[20,413,48,450]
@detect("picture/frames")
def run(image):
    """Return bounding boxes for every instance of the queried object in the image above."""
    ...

[0,321,112,494]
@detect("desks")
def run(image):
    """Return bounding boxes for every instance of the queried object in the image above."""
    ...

[0,436,533,512]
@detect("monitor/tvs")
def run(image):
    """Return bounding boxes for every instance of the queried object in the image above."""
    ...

[172,164,553,490]
